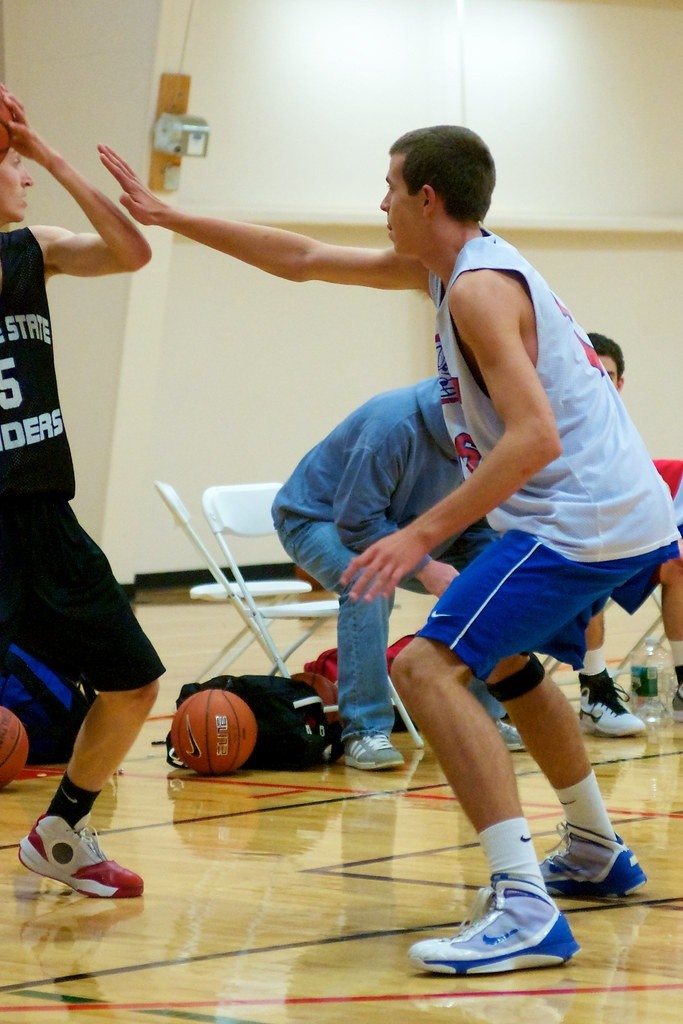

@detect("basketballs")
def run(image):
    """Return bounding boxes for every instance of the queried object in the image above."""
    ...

[0,705,31,790]
[0,82,15,165]
[169,688,261,776]
[290,673,339,731]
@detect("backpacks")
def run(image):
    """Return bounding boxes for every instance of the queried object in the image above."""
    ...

[0,644,98,766]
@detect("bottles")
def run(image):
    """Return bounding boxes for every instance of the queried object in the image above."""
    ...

[629,637,676,730]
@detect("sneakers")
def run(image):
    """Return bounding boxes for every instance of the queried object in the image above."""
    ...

[579,678,646,737]
[344,734,405,769]
[407,872,581,974]
[18,812,144,898]
[496,719,527,752]
[672,684,683,723]
[538,820,647,898]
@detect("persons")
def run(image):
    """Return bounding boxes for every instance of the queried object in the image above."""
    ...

[99,125,682,975]
[0,84,165,898]
[579,334,682,737]
[271,379,524,768]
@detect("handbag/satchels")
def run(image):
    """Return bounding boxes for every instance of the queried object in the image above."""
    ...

[165,674,345,772]
[304,630,422,734]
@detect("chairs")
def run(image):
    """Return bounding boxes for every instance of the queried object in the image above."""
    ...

[155,458,683,758]
